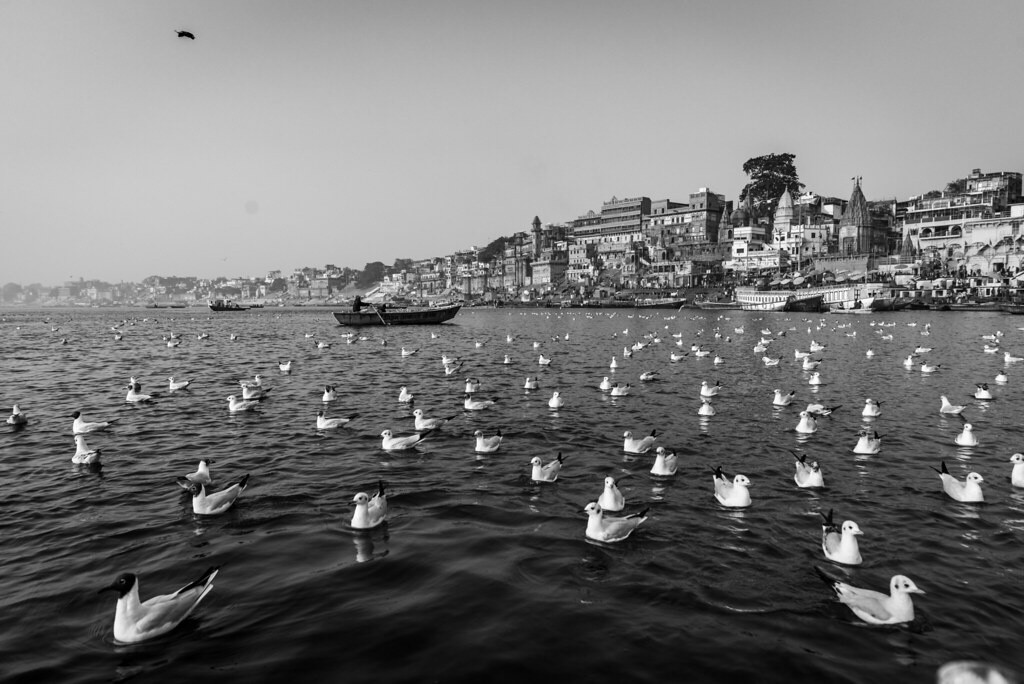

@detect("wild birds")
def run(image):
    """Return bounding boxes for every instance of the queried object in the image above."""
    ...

[174,28,196,41]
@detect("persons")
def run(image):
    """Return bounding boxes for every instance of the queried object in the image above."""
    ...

[352,295,372,312]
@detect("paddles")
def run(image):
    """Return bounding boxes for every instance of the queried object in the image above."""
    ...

[371,304,388,327]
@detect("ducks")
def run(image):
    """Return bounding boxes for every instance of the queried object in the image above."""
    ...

[0,311,1024,644]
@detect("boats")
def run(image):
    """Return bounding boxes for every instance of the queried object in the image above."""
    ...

[543,282,1024,314]
[210,299,251,311]
[330,303,462,326]
